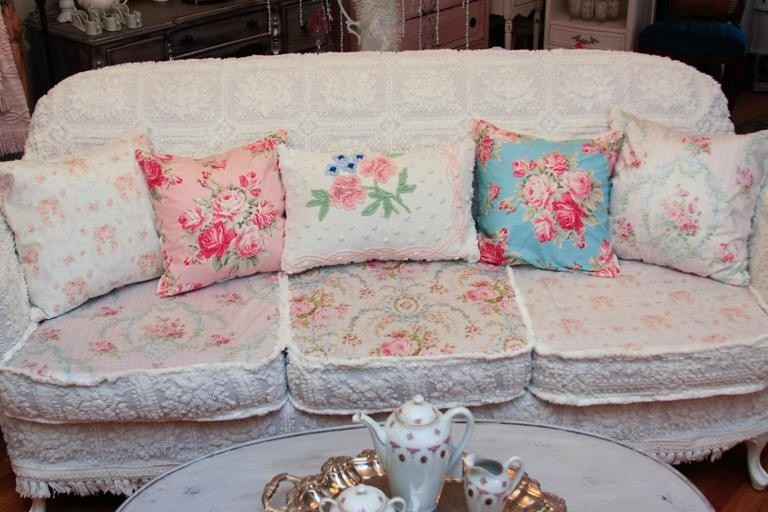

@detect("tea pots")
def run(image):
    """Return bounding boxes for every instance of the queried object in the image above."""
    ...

[319,395,524,512]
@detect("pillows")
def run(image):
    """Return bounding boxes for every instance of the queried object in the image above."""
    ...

[276,139,480,274]
[0,132,165,322]
[608,105,767,288]
[135,128,285,298]
[472,118,623,278]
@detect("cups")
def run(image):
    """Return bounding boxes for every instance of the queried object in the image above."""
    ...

[87,11,142,36]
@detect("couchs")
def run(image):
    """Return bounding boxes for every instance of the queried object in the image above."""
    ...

[0,48,767,494]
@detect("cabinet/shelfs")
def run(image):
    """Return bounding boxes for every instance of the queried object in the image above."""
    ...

[491,0,542,50]
[346,0,488,54]
[543,0,656,50]
[21,1,339,113]
